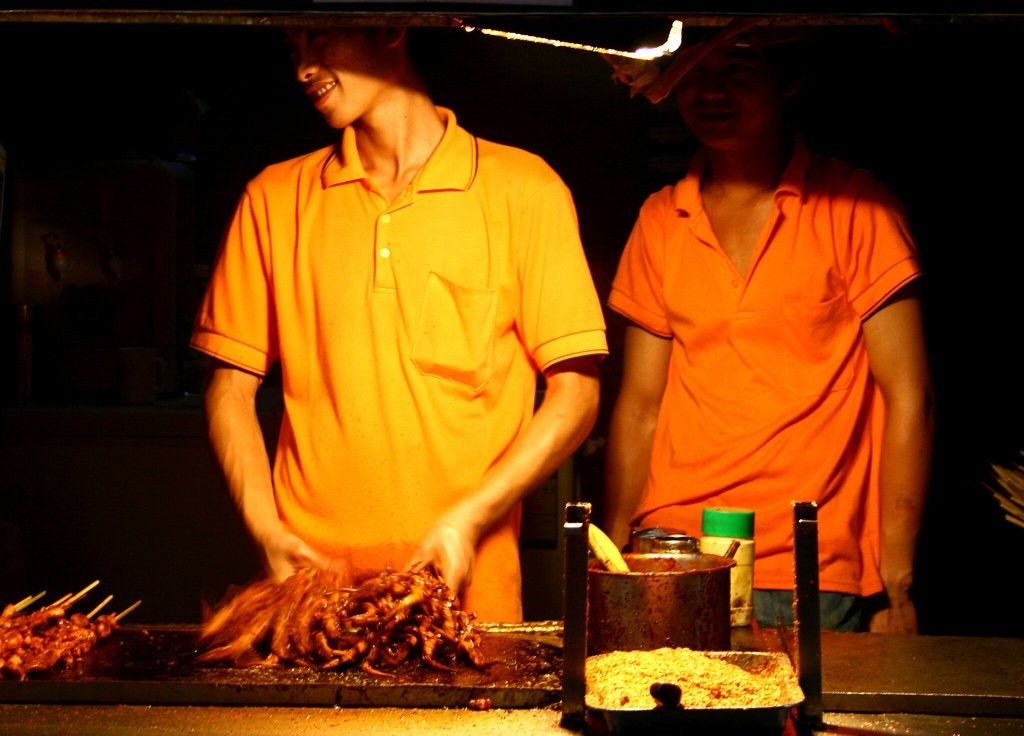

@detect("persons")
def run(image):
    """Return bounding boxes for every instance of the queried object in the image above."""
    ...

[608,51,935,636]
[190,29,610,624]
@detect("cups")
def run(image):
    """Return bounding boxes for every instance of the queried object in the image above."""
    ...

[586,553,736,657]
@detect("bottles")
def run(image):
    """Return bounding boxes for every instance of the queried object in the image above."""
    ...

[630,528,700,554]
[701,507,754,626]
